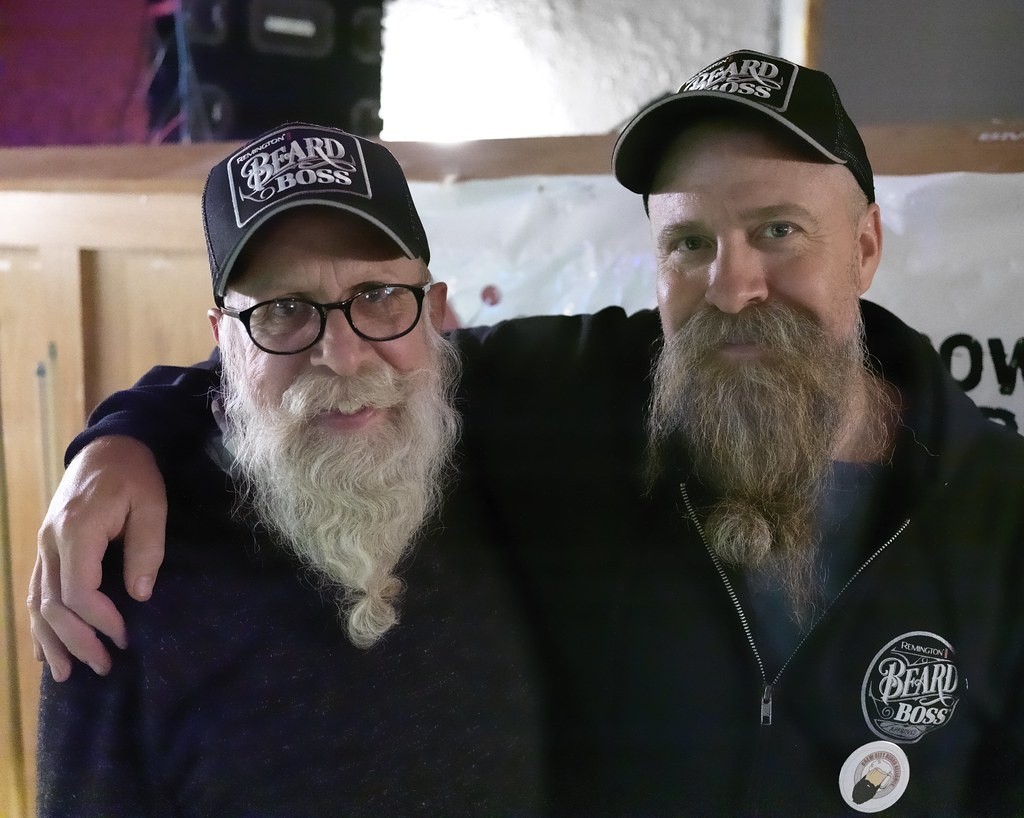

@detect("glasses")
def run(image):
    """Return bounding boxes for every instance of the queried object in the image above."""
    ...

[219,282,432,356]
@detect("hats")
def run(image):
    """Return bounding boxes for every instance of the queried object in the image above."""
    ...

[610,49,875,220]
[202,122,431,309]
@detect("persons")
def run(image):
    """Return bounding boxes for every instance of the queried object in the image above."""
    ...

[35,121,553,818]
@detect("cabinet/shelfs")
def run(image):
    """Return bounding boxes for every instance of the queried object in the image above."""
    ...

[0,119,1024,818]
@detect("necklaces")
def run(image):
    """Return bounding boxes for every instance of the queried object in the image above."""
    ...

[26,48,1024,818]
